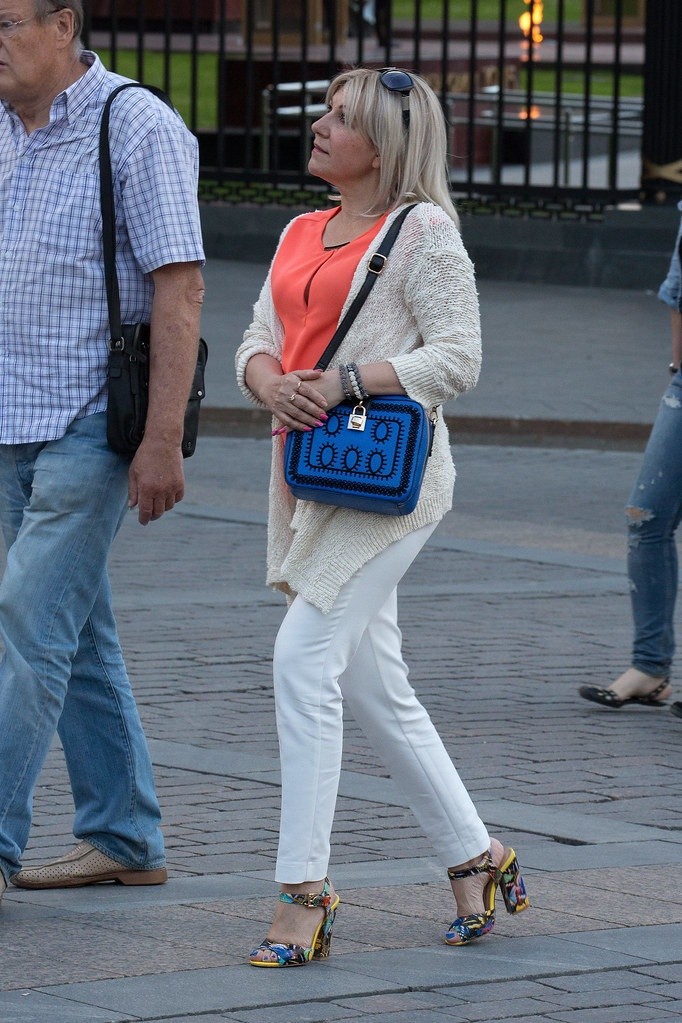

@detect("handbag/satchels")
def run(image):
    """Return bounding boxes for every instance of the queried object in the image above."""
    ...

[106,320,210,458]
[280,395,437,515]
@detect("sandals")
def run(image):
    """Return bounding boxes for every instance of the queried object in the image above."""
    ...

[578,678,673,709]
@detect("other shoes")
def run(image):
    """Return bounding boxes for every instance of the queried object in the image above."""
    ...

[9,840,169,889]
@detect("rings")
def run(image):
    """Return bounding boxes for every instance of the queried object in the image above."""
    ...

[297,382,302,391]
[289,392,297,402]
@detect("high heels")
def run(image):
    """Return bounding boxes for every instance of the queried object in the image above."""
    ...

[443,848,530,945]
[246,877,341,967]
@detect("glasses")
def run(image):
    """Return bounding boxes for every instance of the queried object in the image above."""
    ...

[0,8,59,38]
[381,62,415,132]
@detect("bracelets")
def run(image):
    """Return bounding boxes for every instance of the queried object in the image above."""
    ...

[338,362,369,400]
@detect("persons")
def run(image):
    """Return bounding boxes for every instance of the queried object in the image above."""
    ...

[579,205,682,718]
[233,68,530,966]
[0,0,206,902]
